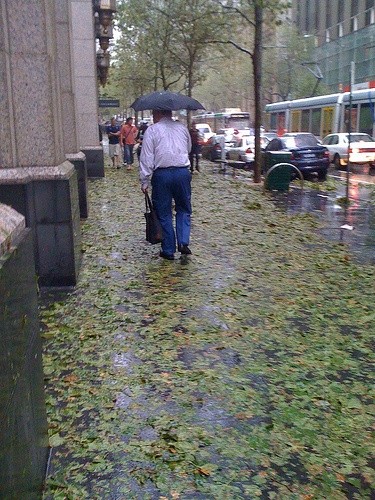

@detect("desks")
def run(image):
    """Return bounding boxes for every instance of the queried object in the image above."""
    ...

[214,160,246,178]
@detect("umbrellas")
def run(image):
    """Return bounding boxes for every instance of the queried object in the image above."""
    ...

[130,91,205,113]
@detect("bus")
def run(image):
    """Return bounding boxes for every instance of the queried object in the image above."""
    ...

[190,112,251,133]
[265,88,375,141]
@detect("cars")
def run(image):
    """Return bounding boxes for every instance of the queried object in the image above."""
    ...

[128,116,154,126]
[193,123,281,161]
[320,132,375,170]
[226,135,271,169]
[260,132,331,180]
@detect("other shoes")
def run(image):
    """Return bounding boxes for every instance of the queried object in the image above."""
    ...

[113,165,116,168]
[127,165,131,170]
[124,163,127,165]
[117,165,120,169]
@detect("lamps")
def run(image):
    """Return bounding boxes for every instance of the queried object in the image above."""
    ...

[93,0,116,34]
[97,51,110,88]
[94,13,114,53]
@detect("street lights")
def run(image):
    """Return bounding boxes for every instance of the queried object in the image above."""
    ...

[302,34,344,92]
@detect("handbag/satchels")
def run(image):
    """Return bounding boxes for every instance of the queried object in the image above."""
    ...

[123,137,126,145]
[144,189,164,244]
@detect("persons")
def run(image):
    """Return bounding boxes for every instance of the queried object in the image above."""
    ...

[101,118,180,170]
[137,109,194,260]
[189,124,201,174]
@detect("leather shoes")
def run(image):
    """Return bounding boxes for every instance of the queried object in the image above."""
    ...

[178,243,191,254]
[160,248,175,260]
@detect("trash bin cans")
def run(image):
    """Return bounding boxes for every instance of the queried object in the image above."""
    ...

[264,151,292,189]
